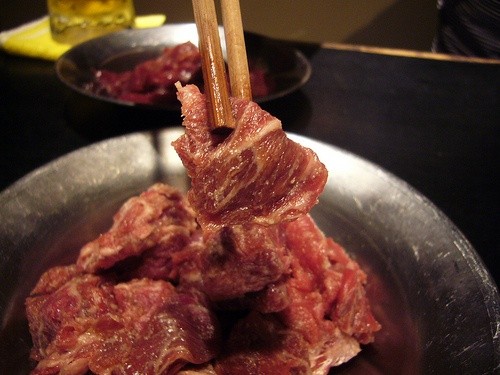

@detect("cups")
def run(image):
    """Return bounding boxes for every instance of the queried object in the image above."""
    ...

[47,0,135,46]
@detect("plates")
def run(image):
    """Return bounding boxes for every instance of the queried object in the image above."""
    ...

[0,126,500,375]
[55,24,312,121]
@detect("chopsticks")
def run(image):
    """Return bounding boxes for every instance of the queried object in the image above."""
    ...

[191,0,252,133]
